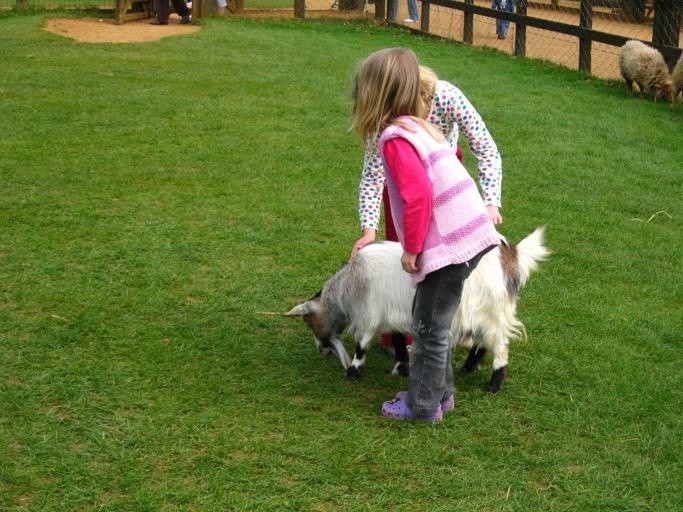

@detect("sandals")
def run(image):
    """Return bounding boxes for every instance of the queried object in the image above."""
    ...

[382,391,454,420]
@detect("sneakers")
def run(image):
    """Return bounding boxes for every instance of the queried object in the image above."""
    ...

[150,11,190,25]
[404,18,419,23]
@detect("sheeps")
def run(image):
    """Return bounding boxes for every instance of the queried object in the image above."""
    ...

[281,224,550,393]
[616,38,677,105]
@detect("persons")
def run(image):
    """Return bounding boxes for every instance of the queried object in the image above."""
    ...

[148,0,190,24]
[386,0,419,23]
[348,62,506,358]
[350,47,502,426]
[490,0,514,39]
